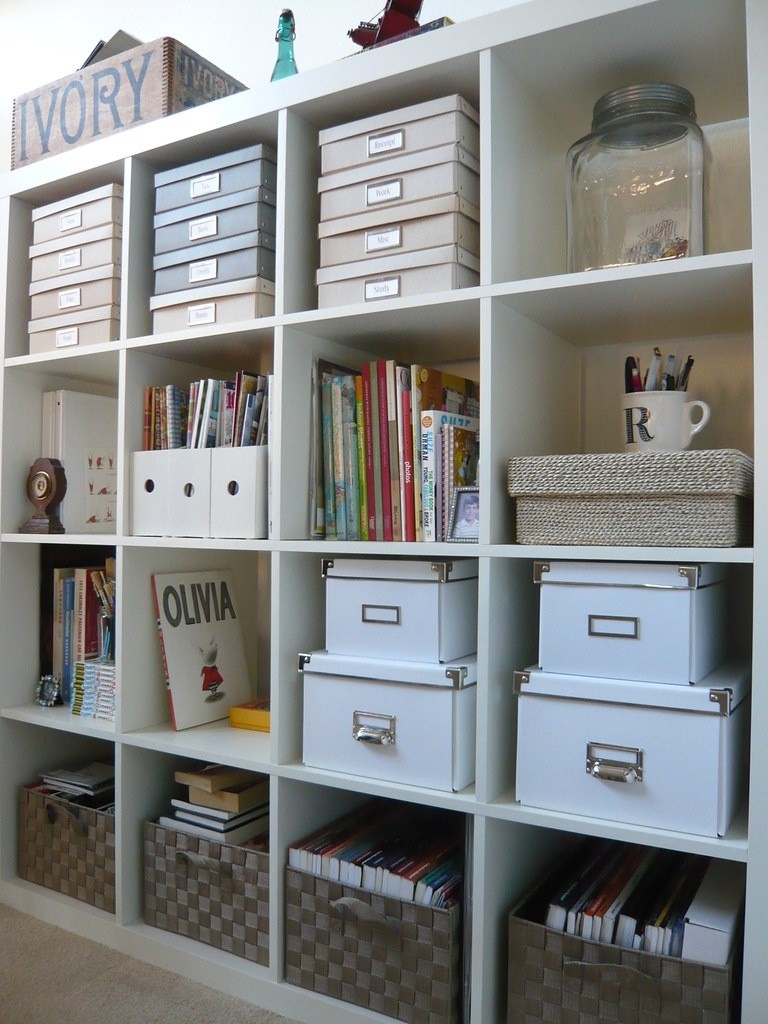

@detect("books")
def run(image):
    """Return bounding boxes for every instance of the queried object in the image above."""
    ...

[39,387,118,532]
[37,753,115,814]
[51,554,117,726]
[286,797,464,909]
[541,841,712,961]
[151,569,253,732]
[142,370,268,452]
[157,762,269,847]
[306,351,479,543]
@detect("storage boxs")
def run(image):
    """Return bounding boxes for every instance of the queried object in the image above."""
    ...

[316,244,480,308]
[152,231,275,297]
[11,36,250,170]
[28,304,121,357]
[29,222,122,282]
[506,447,754,548]
[28,264,121,320]
[17,696,742,1024]
[317,144,480,223]
[318,92,480,176]
[32,183,123,244]
[153,187,276,255]
[129,443,268,540]
[514,652,751,840]
[150,277,275,334]
[322,556,478,663]
[532,561,730,686]
[318,194,481,267]
[298,648,477,793]
[154,144,277,214]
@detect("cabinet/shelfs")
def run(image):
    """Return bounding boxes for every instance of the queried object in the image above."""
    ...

[0,0,768,1024]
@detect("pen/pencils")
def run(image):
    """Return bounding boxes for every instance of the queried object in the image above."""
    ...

[624,347,695,391]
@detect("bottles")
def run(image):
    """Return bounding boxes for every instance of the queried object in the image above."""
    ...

[268,7,298,82]
[562,80,706,273]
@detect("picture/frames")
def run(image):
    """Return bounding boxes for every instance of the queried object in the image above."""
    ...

[446,486,479,544]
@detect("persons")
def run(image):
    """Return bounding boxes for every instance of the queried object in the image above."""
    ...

[451,495,479,537]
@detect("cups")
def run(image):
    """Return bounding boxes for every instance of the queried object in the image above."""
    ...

[619,389,711,453]
